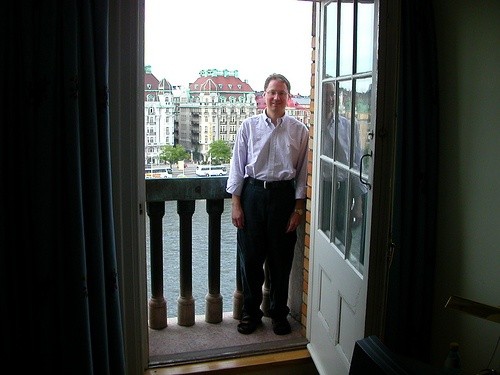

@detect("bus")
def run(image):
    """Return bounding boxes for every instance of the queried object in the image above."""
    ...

[145,167,173,179]
[195,166,228,177]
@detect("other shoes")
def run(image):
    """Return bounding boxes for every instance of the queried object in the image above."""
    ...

[272,318,291,335]
[237,319,262,335]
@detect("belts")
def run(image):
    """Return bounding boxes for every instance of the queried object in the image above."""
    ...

[247,177,294,190]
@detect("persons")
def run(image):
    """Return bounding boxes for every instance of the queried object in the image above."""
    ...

[224,74,309,335]
[321,73,367,235]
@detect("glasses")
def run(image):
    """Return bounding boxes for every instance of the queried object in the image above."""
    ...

[266,91,288,96]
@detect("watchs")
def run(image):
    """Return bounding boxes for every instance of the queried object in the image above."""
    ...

[294,208,303,215]
